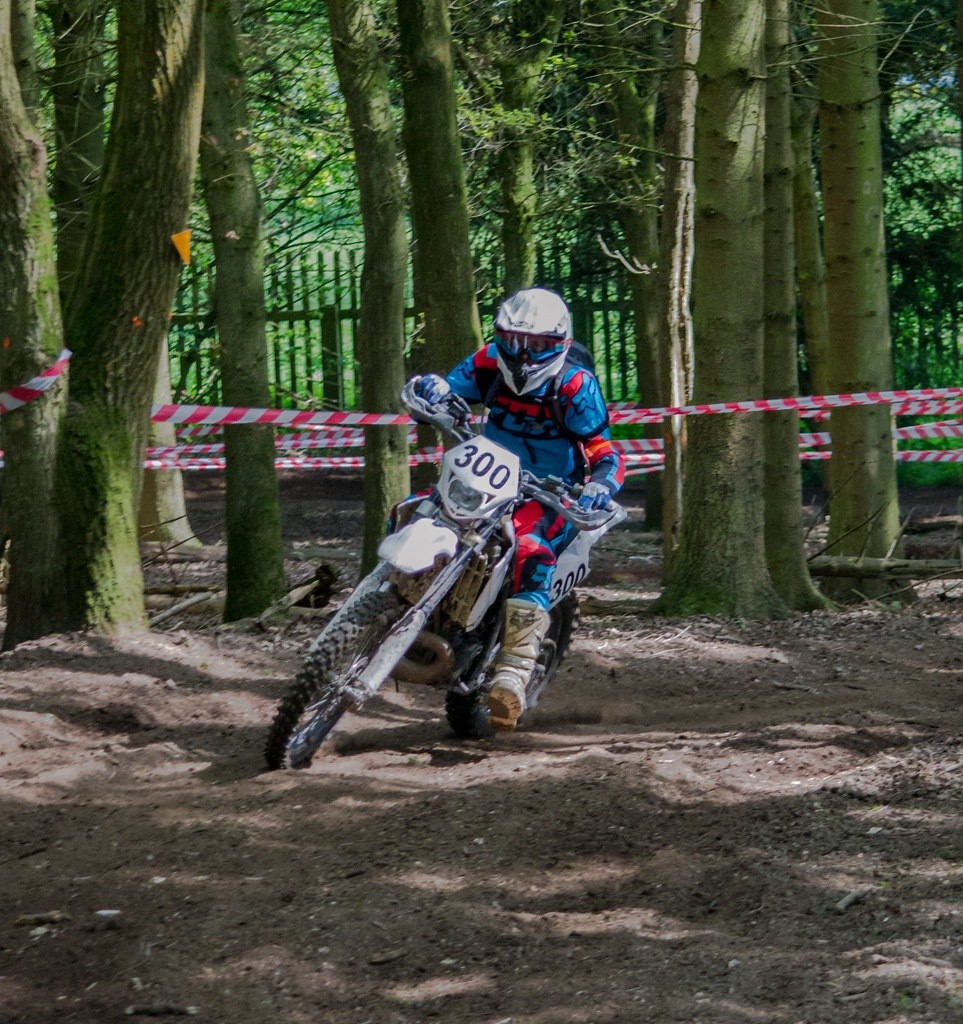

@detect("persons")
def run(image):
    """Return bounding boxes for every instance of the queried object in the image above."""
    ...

[412,289,626,731]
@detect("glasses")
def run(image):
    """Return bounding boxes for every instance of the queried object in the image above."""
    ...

[495,327,571,363]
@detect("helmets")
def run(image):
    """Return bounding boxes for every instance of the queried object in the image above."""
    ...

[495,288,571,396]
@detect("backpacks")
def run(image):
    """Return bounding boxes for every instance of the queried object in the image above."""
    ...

[486,340,594,443]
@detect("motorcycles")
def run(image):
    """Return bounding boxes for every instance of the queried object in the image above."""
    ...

[261,376,630,770]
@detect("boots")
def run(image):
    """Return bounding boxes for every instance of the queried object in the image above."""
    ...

[487,599,551,732]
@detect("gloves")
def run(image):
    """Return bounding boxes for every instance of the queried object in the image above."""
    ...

[419,373,451,406]
[578,481,611,513]
